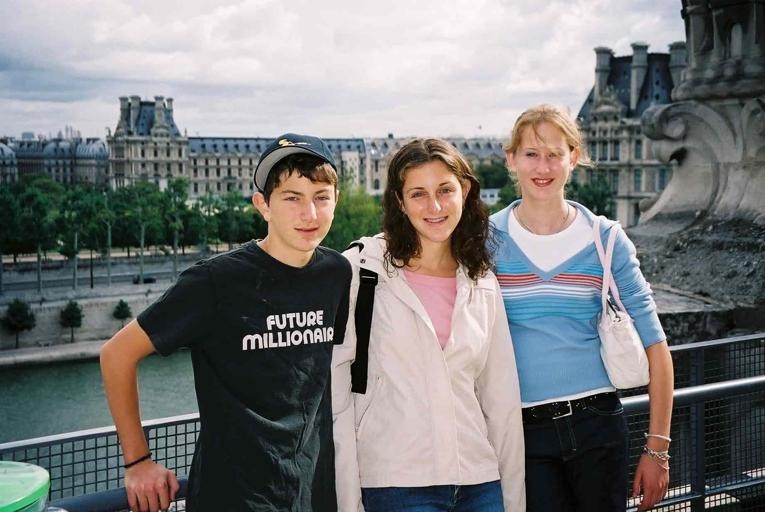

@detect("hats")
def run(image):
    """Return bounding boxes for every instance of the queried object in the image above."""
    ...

[253,133,338,191]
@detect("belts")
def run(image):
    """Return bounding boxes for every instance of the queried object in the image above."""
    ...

[522,392,620,424]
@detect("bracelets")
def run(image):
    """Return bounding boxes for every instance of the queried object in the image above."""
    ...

[641,431,672,444]
[642,445,670,470]
[124,453,153,470]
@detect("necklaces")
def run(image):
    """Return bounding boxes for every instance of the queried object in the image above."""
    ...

[516,204,571,235]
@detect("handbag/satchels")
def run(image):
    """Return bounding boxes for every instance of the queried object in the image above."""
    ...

[593,214,651,390]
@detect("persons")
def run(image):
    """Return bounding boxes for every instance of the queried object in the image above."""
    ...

[485,103,675,511]
[99,133,352,511]
[329,139,525,512]
